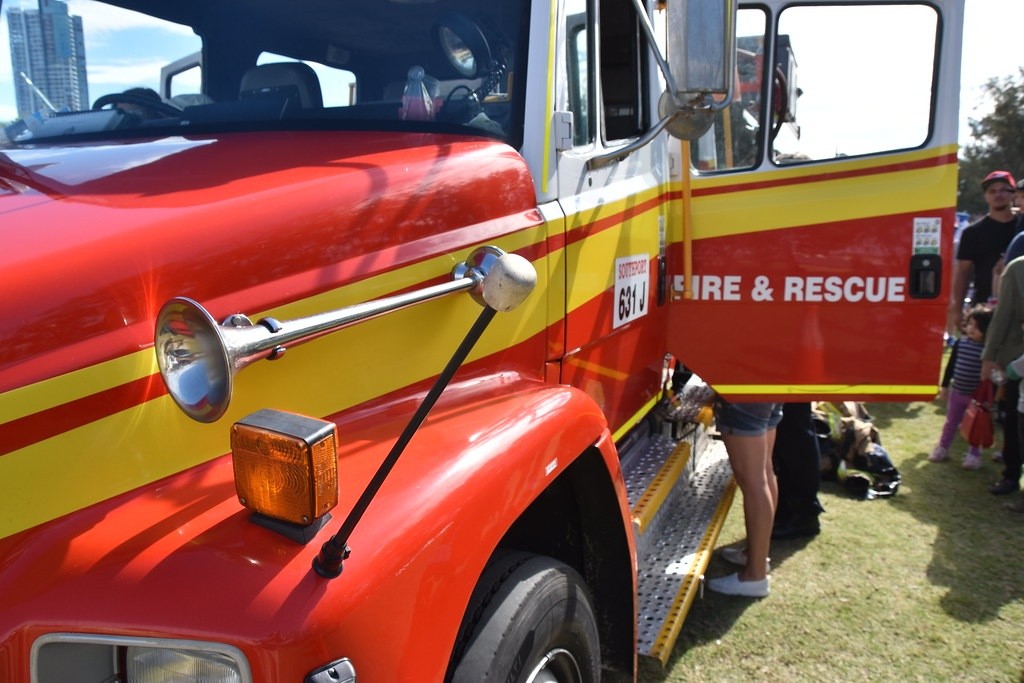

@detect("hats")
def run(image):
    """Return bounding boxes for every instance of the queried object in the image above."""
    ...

[981,170,1017,189]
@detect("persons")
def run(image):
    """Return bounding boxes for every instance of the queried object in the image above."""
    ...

[706,153,826,597]
[928,172,1024,516]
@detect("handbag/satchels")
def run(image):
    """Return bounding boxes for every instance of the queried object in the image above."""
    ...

[960,378,994,448]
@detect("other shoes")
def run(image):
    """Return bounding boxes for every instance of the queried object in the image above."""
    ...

[771,519,820,538]
[1004,503,1024,514]
[992,453,1007,464]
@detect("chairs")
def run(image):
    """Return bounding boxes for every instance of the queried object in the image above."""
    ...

[602,68,635,140]
[238,62,323,109]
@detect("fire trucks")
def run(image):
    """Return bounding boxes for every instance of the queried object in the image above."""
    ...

[0,0,967,679]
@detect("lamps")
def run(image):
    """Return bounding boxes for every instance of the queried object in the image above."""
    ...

[431,10,514,117]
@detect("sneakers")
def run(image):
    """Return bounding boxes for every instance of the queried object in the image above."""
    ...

[707,572,769,597]
[962,452,985,469]
[993,478,1019,495]
[929,446,949,462]
[720,548,771,573]
